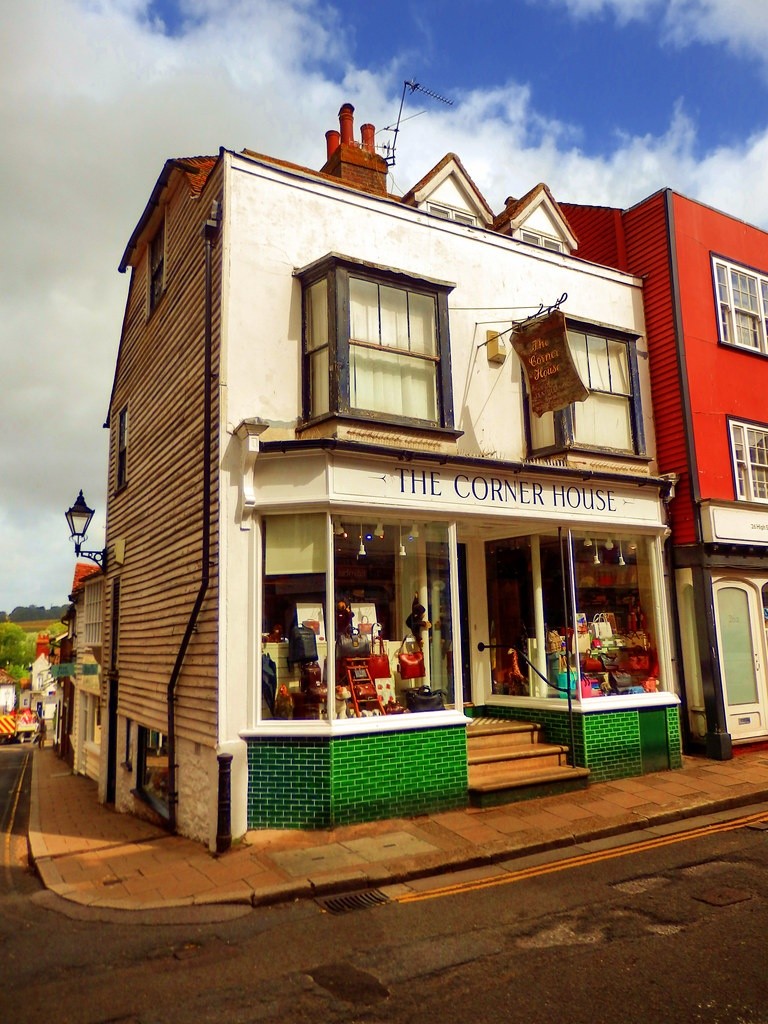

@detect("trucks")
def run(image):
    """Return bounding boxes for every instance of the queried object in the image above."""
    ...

[0,683,40,746]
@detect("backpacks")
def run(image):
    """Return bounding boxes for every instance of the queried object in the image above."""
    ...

[287,623,318,663]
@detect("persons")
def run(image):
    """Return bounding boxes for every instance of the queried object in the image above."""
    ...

[33,718,48,751]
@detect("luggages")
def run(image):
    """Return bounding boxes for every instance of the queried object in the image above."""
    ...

[299,661,322,692]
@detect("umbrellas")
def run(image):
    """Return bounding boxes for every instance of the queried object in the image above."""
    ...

[262,651,277,719]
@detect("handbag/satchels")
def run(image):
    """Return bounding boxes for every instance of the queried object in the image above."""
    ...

[336,627,370,659]
[358,616,372,635]
[405,685,448,713]
[354,682,377,700]
[366,636,391,678]
[301,618,320,635]
[544,613,662,700]
[397,634,426,680]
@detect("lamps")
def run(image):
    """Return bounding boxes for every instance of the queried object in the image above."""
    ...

[618,534,626,565]
[374,518,385,537]
[409,519,420,537]
[65,489,109,572]
[628,534,638,550]
[606,532,614,550]
[593,533,600,564]
[332,515,345,534]
[399,520,406,556]
[359,521,366,555]
[584,531,592,546]
